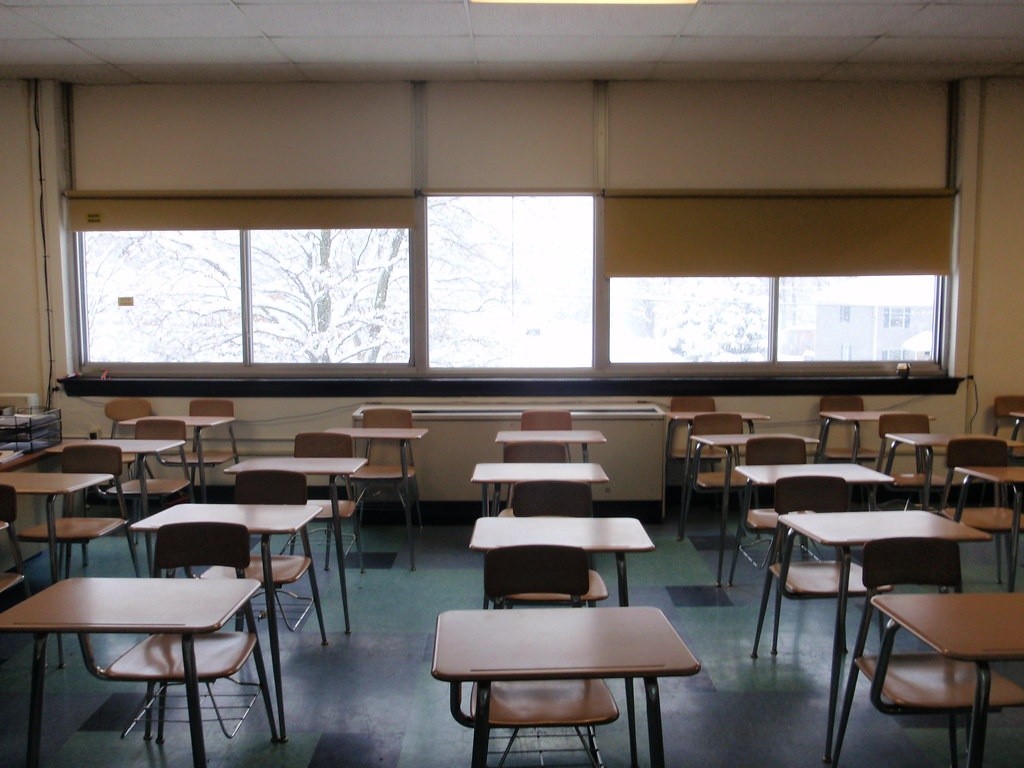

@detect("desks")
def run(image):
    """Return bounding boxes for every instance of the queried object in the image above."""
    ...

[469,461,609,517]
[223,456,367,635]
[884,432,1023,512]
[814,409,935,464]
[41,438,195,518]
[431,605,700,768]
[680,434,820,587]
[0,472,115,585]
[127,502,329,745]
[118,415,236,505]
[0,576,278,767]
[493,430,607,463]
[468,517,656,608]
[661,412,770,541]
[728,462,894,588]
[772,509,992,762]
[322,426,428,572]
[952,463,1023,594]
[869,593,1023,768]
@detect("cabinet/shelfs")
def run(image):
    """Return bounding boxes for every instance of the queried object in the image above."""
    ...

[0,440,88,573]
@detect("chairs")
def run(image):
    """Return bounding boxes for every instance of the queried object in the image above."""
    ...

[0,396,1023,768]
